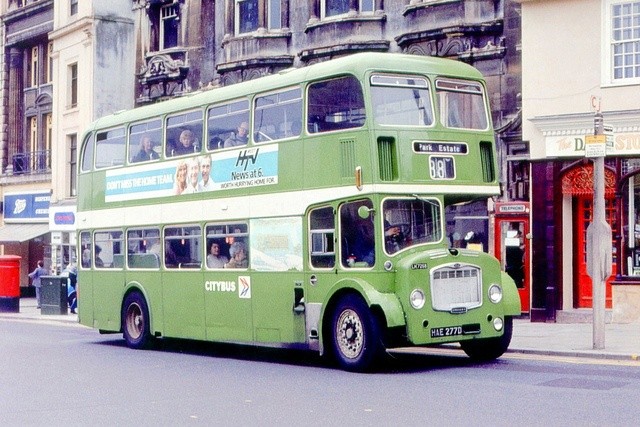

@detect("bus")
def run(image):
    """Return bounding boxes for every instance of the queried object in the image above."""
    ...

[74,50,523,372]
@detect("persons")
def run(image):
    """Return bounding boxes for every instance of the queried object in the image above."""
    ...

[181,160,204,194]
[358,205,401,264]
[165,139,175,156]
[198,154,216,192]
[176,130,195,154]
[207,240,229,269]
[131,136,160,161]
[171,161,188,195]
[28,260,49,308]
[226,241,249,269]
[150,232,180,267]
[224,121,248,148]
